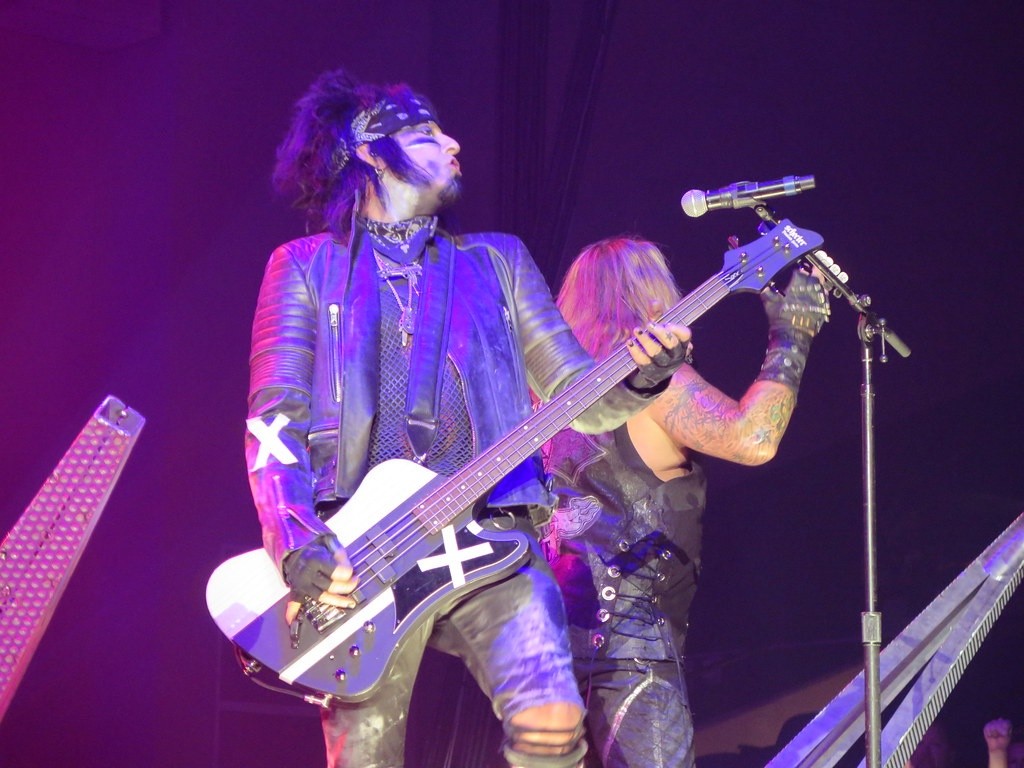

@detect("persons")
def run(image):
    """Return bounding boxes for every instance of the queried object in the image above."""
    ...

[983,718,1024,768]
[532,235,830,768]
[244,64,694,767]
[910,716,950,768]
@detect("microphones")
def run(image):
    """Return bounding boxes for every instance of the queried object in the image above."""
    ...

[681,175,816,217]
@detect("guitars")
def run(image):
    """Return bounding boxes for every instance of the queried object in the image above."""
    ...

[204,217,825,708]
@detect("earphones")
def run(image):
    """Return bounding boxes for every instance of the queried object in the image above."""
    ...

[370,149,378,156]
